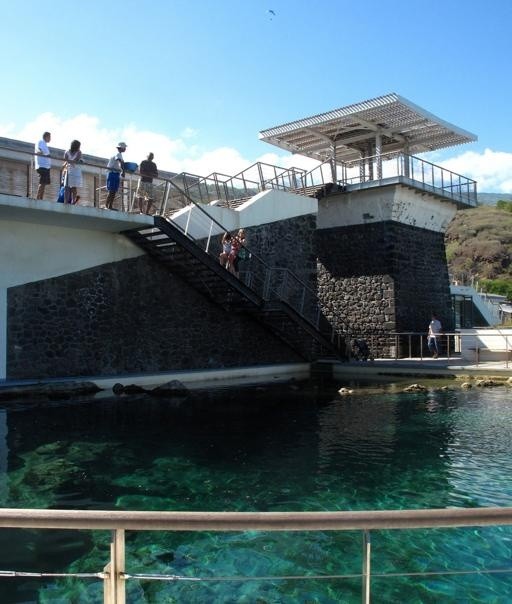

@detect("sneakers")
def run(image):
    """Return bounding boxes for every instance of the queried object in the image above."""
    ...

[433,354,437,358]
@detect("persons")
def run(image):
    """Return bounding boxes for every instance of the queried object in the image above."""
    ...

[57,139,83,205]
[426,313,441,358]
[35,132,51,200]
[105,142,126,211]
[136,153,158,216]
[219,229,247,279]
[350,339,369,362]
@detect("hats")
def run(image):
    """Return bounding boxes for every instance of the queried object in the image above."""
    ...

[116,141,126,149]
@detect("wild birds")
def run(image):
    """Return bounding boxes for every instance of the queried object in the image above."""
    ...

[269,9,276,16]
